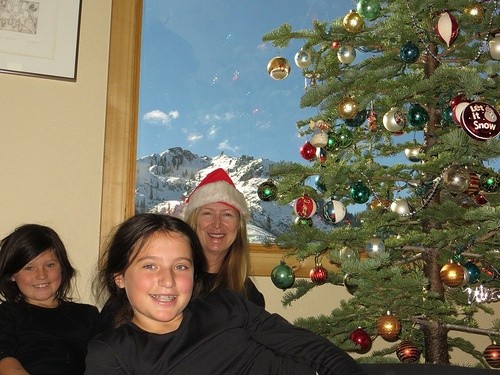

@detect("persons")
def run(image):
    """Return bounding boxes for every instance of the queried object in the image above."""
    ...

[101,167,266,337]
[0,224,104,375]
[83,212,371,375]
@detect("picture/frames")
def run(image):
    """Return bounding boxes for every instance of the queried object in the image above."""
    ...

[99,0,500,279]
[0,0,83,83]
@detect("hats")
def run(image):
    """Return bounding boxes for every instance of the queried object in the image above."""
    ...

[182,167,250,225]
